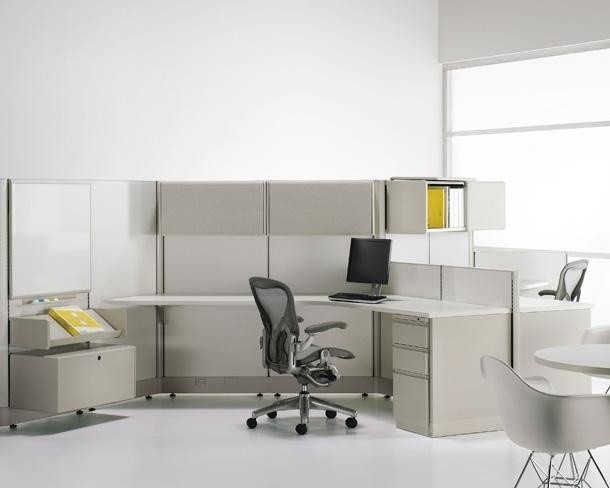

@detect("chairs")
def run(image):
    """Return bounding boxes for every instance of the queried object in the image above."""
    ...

[581,324,610,344]
[479,354,610,488]
[539,259,589,302]
[248,276,359,434]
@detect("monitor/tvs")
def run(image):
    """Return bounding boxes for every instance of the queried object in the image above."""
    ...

[346,238,393,295]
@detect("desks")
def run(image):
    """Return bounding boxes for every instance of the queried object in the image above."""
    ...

[104,294,511,439]
[535,344,610,394]
[518,279,594,397]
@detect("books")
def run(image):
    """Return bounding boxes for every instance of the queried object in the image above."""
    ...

[428,186,465,229]
[48,305,105,337]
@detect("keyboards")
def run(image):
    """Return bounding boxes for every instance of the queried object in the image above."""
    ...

[329,293,387,303]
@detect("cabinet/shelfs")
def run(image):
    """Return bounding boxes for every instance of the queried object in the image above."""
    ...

[154,179,377,237]
[387,179,506,236]
